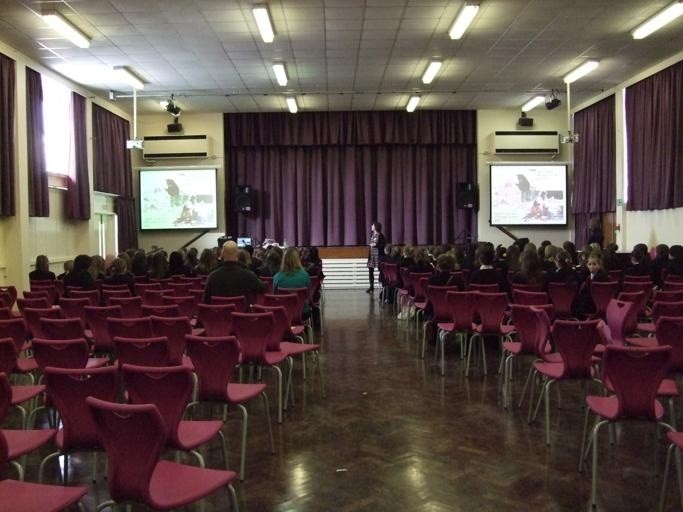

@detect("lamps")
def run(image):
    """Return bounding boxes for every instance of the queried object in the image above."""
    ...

[41,10,92,49]
[252,4,275,43]
[286,95,298,113]
[520,94,545,112]
[166,94,180,115]
[545,91,559,111]
[404,94,421,113]
[421,58,442,84]
[448,1,481,41]
[113,66,145,91]
[561,58,600,85]
[630,1,683,40]
[273,61,288,87]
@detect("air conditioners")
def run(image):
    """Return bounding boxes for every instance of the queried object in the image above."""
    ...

[494,131,561,155]
[143,135,208,159]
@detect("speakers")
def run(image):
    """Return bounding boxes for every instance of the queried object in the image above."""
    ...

[232,185,254,213]
[456,181,476,209]
[166,123,182,133]
[519,118,533,126]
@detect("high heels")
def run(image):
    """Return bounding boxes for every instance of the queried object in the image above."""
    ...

[366,287,376,294]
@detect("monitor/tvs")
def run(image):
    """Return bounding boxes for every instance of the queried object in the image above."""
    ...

[236,236,252,247]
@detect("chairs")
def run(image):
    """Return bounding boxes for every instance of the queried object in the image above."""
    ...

[0,372,46,431]
[625,316,683,378]
[0,271,85,359]
[636,302,683,338]
[265,293,300,326]
[502,305,551,408]
[596,321,679,431]
[500,303,554,372]
[189,269,206,336]
[205,273,273,314]
[166,276,189,353]
[115,280,166,338]
[44,366,121,484]
[500,291,548,373]
[306,276,320,334]
[623,281,653,308]
[86,277,114,356]
[231,311,296,422]
[382,262,507,380]
[579,269,621,318]
[1,429,57,483]
[197,303,244,385]
[513,284,545,292]
[267,320,327,411]
[32,339,107,431]
[585,345,678,468]
[529,320,600,446]
[548,281,576,326]
[278,287,316,362]
[652,268,683,302]
[0,338,40,413]
[582,344,683,509]
[623,274,652,282]
[84,397,238,511]
[113,336,197,419]
[122,364,229,470]
[519,307,600,424]
[184,335,276,483]
[593,299,633,361]
[616,290,645,345]
[0,478,89,512]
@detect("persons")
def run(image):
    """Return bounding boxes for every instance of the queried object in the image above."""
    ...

[201,236,322,303]
[28,255,56,279]
[173,195,197,223]
[523,191,548,219]
[58,247,199,278]
[366,220,386,293]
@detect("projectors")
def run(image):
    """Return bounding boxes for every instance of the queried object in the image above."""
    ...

[560,136,579,143]
[126,139,144,150]
